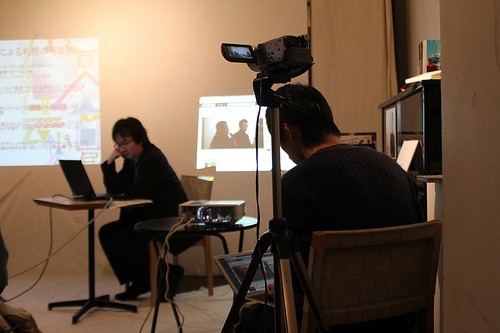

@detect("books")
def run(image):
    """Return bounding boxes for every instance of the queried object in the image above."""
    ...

[418,39,442,76]
[405,69,442,84]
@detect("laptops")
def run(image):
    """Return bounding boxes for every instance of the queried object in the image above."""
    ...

[214,248,275,307]
[60,160,132,200]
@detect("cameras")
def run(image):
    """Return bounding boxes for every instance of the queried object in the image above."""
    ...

[221,34,314,83]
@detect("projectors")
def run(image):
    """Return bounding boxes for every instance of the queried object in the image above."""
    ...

[179,199,246,222]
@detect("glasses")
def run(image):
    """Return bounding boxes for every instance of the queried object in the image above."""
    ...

[114,140,133,150]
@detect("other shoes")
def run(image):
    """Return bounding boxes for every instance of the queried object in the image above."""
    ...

[157,263,185,305]
[114,278,151,301]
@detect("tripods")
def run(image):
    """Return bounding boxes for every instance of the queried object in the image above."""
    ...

[222,75,326,333]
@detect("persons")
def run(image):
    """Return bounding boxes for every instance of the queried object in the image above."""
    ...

[231,119,252,148]
[98,117,189,302]
[233,82,425,333]
[210,121,234,149]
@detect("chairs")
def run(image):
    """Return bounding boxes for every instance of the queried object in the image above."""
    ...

[150,175,214,306]
[299,220,443,333]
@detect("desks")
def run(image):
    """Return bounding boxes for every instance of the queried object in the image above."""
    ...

[416,175,443,333]
[134,217,258,333]
[33,198,147,325]
